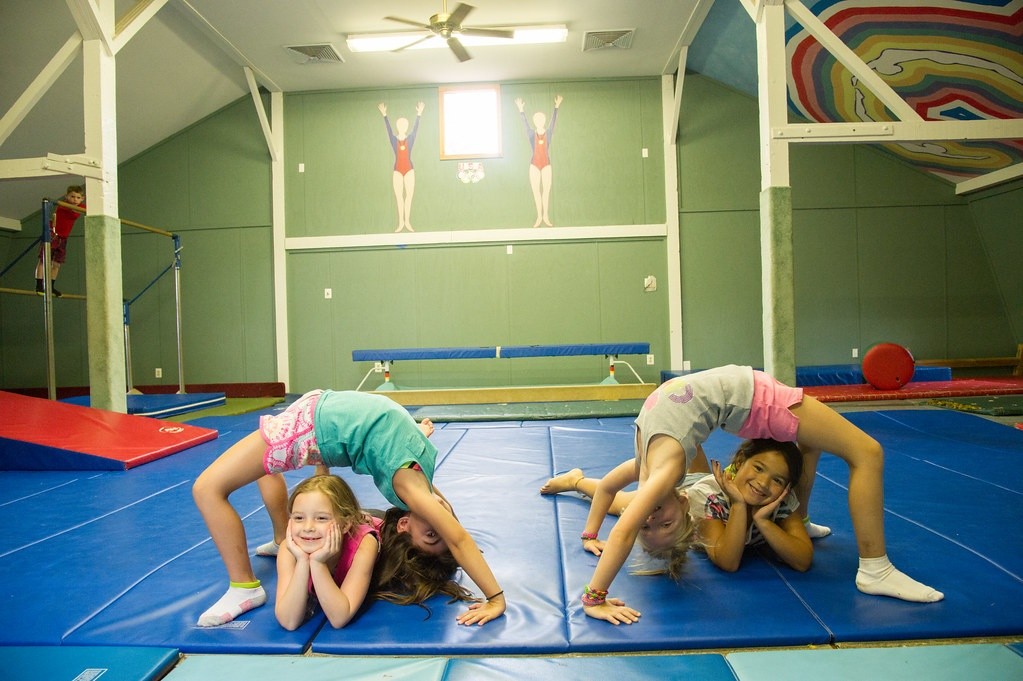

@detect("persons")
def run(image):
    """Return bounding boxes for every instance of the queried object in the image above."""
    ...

[539,437,814,572]
[583,364,944,626]
[35,186,86,296]
[275,418,435,631]
[192,389,506,627]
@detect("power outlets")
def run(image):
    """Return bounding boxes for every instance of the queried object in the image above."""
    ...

[646,354,655,364]
[374,363,383,373]
[155,367,162,380]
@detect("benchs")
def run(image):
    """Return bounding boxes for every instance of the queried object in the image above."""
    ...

[351,343,649,390]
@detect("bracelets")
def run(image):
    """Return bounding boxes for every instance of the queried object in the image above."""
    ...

[581,585,608,606]
[581,532,597,540]
[486,590,504,600]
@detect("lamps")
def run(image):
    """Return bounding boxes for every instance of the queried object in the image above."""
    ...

[346,24,568,53]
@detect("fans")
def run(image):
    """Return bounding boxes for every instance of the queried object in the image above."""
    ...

[383,0,515,62]
